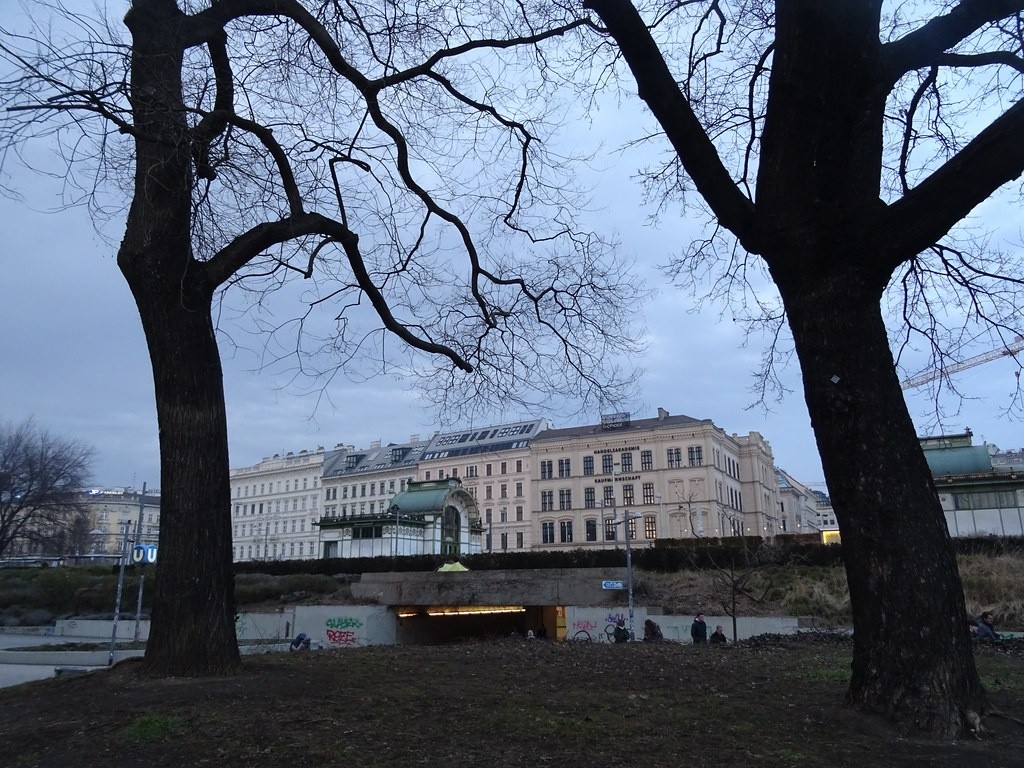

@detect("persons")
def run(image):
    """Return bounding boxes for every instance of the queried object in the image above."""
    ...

[614,619,629,644]
[290,633,311,653]
[969,611,1000,640]
[643,619,662,641]
[691,612,707,643]
[709,625,727,644]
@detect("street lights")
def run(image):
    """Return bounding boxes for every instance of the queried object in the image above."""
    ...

[612,468,618,549]
